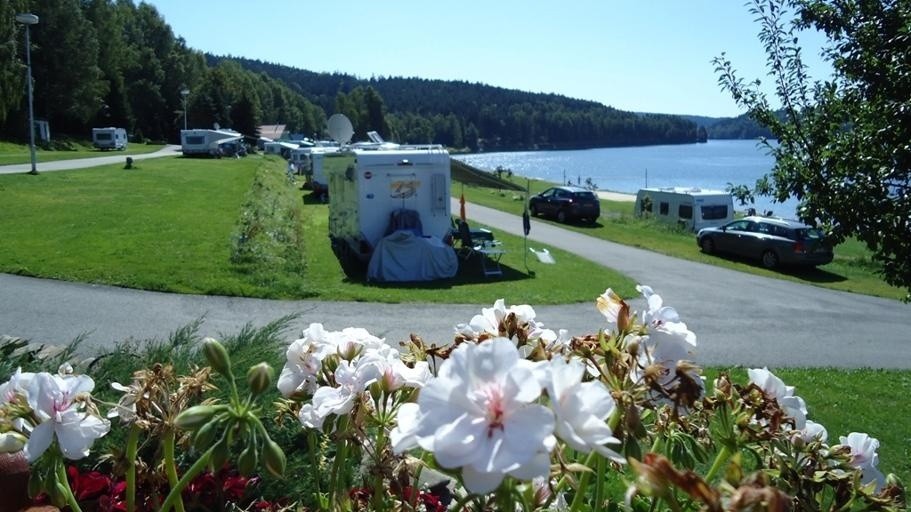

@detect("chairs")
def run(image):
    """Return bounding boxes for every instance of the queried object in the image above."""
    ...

[455,218,482,260]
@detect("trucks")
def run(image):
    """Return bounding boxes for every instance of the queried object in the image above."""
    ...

[93,127,128,150]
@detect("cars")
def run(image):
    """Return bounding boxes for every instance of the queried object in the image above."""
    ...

[528,185,600,224]
[696,215,834,275]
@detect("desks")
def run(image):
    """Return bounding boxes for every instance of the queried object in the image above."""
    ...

[479,249,505,277]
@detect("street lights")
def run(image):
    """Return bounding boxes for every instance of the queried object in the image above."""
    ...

[15,11,39,174]
[180,89,190,130]
